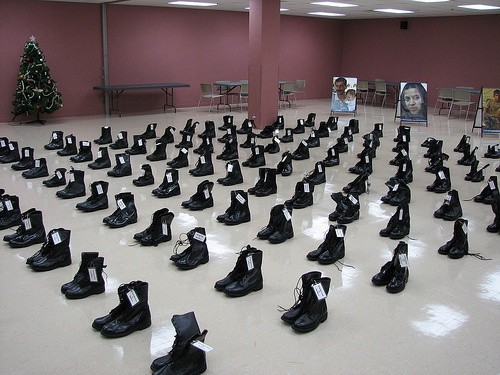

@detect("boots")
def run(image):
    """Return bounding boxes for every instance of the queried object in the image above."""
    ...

[153,329,208,375]
[26,228,64,266]
[293,277,331,332]
[306,223,356,272]
[437,219,469,260]
[92,281,143,330]
[386,243,409,294]
[3,208,46,248]
[372,241,405,287]
[61,252,98,294]
[65,257,108,300]
[101,282,151,338]
[276,271,321,324]
[0,111,500,231]
[170,227,209,270]
[379,203,410,239]
[215,245,257,290]
[257,204,293,244]
[30,230,71,271]
[150,311,201,372]
[133,208,175,246]
[223,249,264,297]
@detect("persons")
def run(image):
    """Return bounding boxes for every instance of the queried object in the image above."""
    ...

[483,89,500,130]
[331,78,348,111]
[400,82,426,120]
[344,89,356,111]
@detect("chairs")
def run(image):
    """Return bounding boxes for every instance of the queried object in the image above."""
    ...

[197,80,307,111]
[433,86,475,120]
[356,80,400,110]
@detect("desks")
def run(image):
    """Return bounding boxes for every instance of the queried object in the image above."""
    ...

[214,82,291,112]
[438,90,481,116]
[93,82,190,118]
[366,81,400,109]
